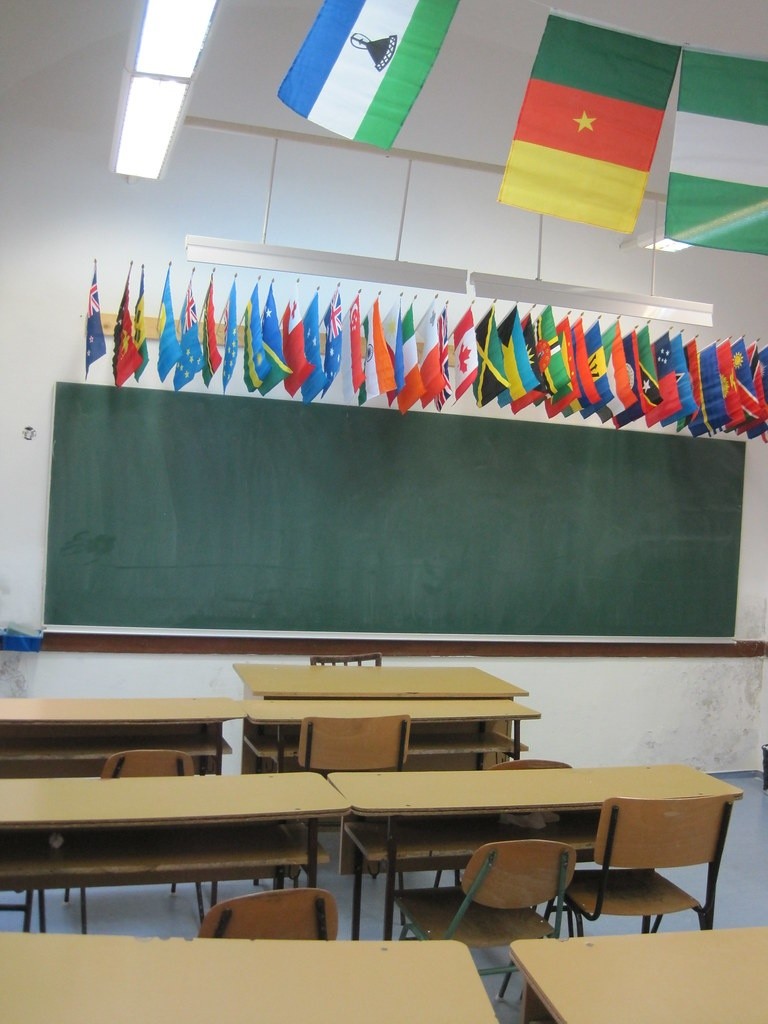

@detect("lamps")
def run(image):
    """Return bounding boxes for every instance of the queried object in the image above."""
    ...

[105,0,222,181]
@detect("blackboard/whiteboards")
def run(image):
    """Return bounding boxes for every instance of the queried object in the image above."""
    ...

[37,378,751,644]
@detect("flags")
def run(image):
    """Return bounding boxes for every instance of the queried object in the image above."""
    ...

[86,262,106,379]
[112,265,149,387]
[499,7,767,254]
[278,0,461,150]
[157,270,768,445]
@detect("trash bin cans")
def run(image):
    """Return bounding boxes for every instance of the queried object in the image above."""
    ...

[761,744,768,790]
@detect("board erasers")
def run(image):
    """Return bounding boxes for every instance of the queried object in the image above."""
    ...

[6,621,39,635]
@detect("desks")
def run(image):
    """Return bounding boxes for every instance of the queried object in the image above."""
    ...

[507,927,768,1024]
[243,699,541,773]
[1,698,248,775]
[1,932,502,1024]
[0,771,351,887]
[328,762,745,940]
[233,662,531,773]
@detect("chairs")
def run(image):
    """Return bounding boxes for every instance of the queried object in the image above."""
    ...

[1,650,768,1024]
